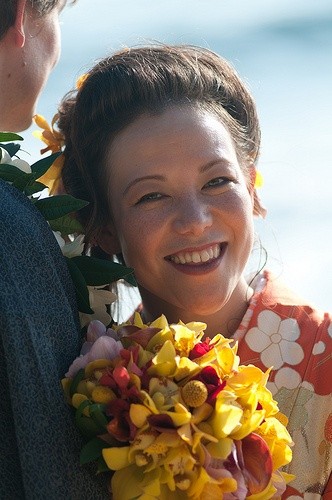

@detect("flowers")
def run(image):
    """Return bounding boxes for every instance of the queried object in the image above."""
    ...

[65,312,296,500]
[0,74,263,328]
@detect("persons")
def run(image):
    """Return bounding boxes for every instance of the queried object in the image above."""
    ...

[61,45,332,500]
[0,0,111,500]
